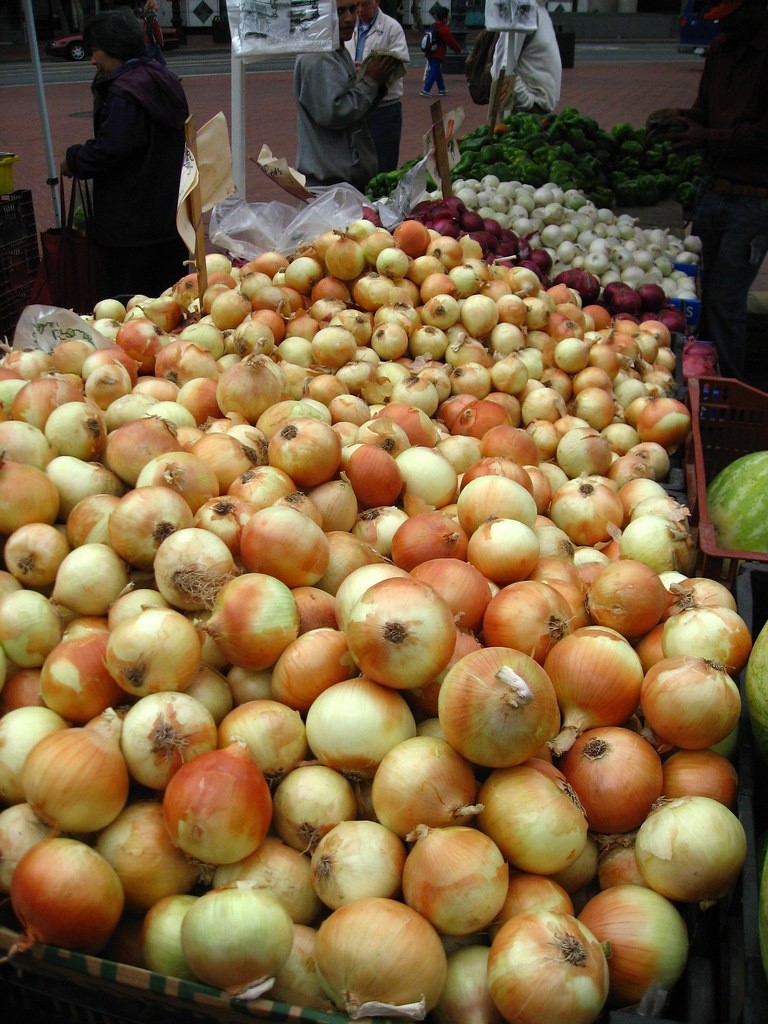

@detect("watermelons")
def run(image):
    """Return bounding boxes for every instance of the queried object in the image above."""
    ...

[745,619,768,762]
[706,452,768,577]
[758,845,768,974]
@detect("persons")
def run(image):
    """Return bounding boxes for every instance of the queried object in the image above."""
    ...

[60,9,190,301]
[490,0,562,116]
[646,0,768,384]
[294,0,411,197]
[411,0,424,34]
[395,2,407,24]
[142,0,167,66]
[419,7,461,97]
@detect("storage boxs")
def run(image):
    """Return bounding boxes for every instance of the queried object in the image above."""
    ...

[715,562,768,1024]
[686,374,768,598]
[0,151,41,357]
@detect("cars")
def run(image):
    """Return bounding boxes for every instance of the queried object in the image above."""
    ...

[47,27,180,61]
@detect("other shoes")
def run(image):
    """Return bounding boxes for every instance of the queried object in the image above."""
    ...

[438,90,448,95]
[420,91,433,98]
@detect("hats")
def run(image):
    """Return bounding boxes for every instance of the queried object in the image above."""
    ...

[697,0,757,20]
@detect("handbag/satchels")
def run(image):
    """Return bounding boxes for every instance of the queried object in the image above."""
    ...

[30,168,102,317]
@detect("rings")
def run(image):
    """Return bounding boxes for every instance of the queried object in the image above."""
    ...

[61,169,65,173]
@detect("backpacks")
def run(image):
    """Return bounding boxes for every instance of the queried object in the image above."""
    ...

[420,23,447,54]
[464,12,539,105]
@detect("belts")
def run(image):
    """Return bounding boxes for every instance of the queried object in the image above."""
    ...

[709,176,768,198]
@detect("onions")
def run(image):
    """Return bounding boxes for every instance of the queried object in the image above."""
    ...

[0,175,751,1024]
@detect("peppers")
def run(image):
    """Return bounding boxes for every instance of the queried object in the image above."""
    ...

[364,107,704,210]
